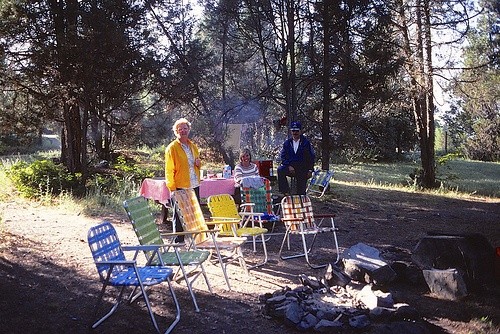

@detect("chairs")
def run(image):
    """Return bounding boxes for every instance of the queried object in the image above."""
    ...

[278,195,340,269]
[87,221,181,334]
[307,169,333,199]
[170,189,249,291]
[205,194,268,271]
[239,177,292,253]
[122,197,215,313]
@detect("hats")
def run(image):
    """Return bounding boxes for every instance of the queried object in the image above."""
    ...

[289,121,302,131]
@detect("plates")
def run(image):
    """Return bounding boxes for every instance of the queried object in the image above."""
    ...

[212,178,226,180]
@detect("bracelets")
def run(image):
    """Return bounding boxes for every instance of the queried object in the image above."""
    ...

[309,170,313,172]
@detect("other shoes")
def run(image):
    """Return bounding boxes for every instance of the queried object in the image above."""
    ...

[277,192,289,198]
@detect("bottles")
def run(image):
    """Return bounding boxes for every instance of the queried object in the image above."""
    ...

[207,168,210,180]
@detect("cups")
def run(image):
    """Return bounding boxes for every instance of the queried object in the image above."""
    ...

[217,173,222,178]
[200,170,203,180]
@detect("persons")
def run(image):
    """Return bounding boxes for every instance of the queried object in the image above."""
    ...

[233,148,259,191]
[278,121,314,194]
[165,118,201,246]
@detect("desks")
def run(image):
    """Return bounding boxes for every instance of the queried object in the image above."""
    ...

[139,176,236,233]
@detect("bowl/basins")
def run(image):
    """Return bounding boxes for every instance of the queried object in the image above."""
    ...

[208,174,214,179]
